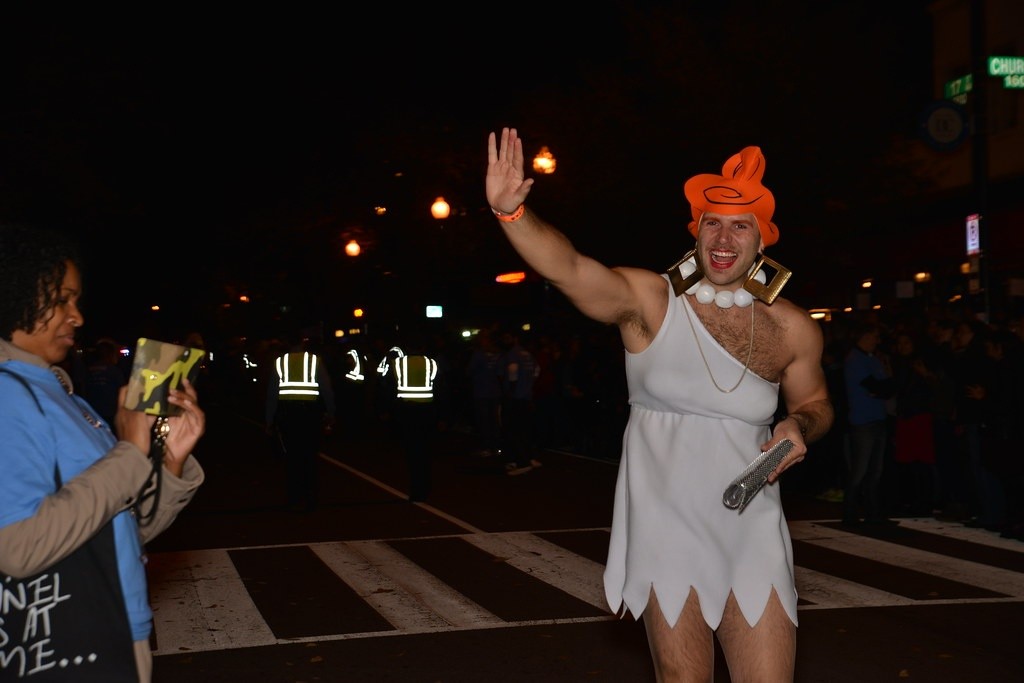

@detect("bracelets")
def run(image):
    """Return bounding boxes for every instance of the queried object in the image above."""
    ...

[783,417,807,438]
[490,204,528,223]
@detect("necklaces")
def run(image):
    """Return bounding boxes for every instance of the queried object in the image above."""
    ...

[52,369,103,430]
[680,294,755,393]
[677,250,767,309]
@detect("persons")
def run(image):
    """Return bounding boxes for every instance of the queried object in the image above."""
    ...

[484,128,833,683]
[63,316,1024,512]
[0,246,206,683]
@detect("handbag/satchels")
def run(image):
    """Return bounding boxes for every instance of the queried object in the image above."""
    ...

[0,519,140,683]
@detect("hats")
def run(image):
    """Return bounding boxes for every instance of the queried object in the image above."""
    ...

[684,145,781,248]
